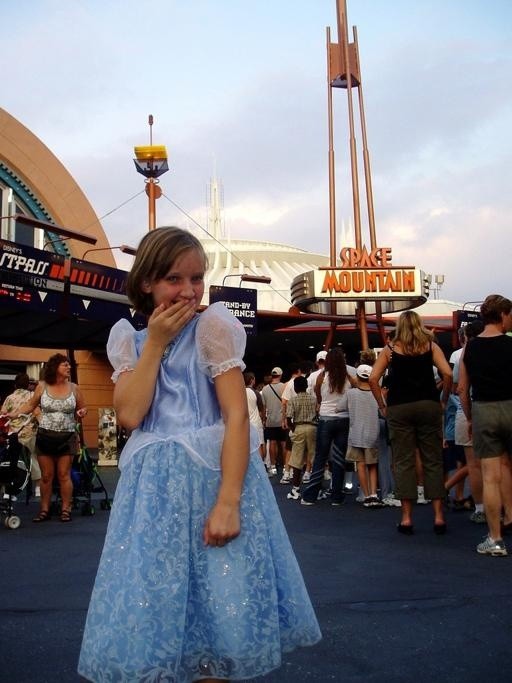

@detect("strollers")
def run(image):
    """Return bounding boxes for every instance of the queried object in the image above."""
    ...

[53,424,114,515]
[1,414,32,531]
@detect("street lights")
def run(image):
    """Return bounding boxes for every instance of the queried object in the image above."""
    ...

[119,115,170,257]
[427,273,445,299]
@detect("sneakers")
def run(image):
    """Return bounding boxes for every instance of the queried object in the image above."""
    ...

[356,485,432,506]
[470,510,487,522]
[268,467,333,504]
[477,536,508,556]
[448,499,464,508]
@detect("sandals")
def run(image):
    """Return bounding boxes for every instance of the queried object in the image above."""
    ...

[32,510,51,522]
[61,509,71,521]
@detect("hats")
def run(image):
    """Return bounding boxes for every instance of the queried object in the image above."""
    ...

[357,364,373,378]
[317,350,328,361]
[271,366,283,375]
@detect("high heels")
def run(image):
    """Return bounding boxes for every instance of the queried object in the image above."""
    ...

[396,522,414,534]
[433,519,448,535]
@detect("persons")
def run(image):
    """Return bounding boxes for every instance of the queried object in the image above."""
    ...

[76,229,324,682]
[2,374,41,497]
[245,293,512,555]
[3,353,88,521]
